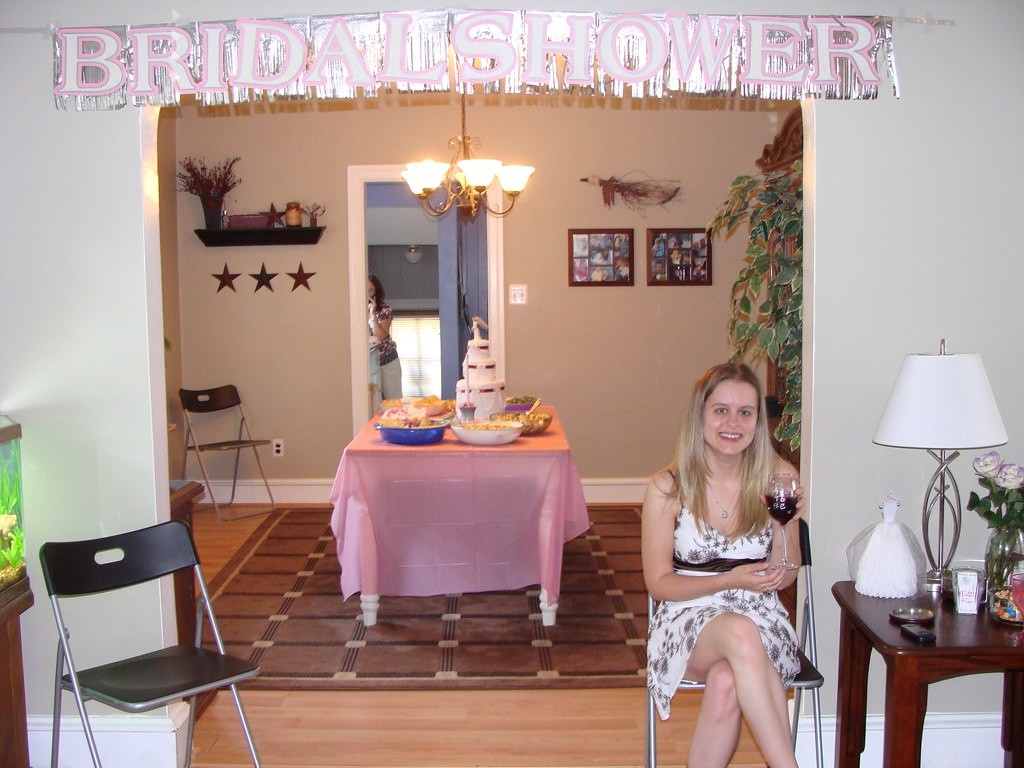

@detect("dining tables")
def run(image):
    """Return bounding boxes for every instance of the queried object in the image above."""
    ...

[326,399,589,631]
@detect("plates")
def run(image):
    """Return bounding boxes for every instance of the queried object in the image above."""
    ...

[889,608,935,624]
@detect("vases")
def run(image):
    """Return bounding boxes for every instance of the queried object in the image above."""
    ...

[984,525,1024,629]
[201,191,228,230]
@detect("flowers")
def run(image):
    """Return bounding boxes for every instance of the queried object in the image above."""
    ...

[169,151,246,196]
[965,450,1024,588]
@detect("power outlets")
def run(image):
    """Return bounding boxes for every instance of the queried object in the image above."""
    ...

[272,439,284,457]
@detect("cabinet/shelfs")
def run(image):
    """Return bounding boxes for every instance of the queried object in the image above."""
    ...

[168,479,205,700]
[0,589,36,768]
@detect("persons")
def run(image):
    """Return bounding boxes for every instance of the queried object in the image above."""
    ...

[367,274,402,401]
[640,362,806,767]
[572,233,708,285]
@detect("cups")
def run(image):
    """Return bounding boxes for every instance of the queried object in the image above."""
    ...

[952,566,985,616]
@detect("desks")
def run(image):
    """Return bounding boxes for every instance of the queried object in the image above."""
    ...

[828,578,1024,768]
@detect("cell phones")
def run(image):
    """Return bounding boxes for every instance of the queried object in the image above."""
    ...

[901,624,937,643]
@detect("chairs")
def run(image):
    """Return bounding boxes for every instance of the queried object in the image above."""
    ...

[647,516,824,768]
[177,384,276,522]
[40,518,268,768]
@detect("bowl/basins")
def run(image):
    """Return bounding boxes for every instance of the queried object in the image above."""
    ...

[489,411,553,434]
[374,419,450,445]
[505,402,540,412]
[450,420,524,445]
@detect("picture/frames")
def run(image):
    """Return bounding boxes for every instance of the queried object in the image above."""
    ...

[566,227,636,289]
[644,227,714,287]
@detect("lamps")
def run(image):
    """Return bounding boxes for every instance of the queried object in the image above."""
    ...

[871,335,1009,591]
[405,91,537,219]
[402,246,424,265]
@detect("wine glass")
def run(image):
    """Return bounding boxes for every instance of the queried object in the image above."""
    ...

[766,474,799,574]
[1011,574,1024,640]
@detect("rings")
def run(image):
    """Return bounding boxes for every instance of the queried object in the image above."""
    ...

[767,587,772,595]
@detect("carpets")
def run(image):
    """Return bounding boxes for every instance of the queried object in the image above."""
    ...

[201,501,653,695]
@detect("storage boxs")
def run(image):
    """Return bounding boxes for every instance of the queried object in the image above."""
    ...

[0,411,34,605]
[229,210,269,229]
